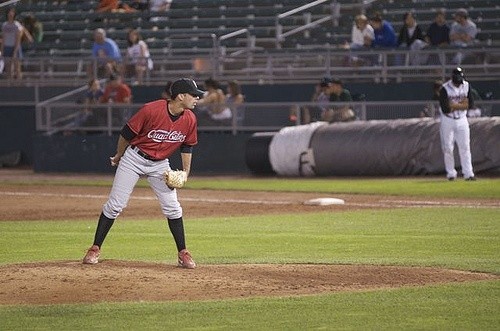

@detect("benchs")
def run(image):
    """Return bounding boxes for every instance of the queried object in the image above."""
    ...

[0,0,500,84]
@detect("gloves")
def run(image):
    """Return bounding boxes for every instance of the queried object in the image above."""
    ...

[166,170,188,188]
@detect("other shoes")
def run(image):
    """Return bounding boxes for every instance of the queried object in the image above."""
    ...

[466,176,477,181]
[449,176,455,181]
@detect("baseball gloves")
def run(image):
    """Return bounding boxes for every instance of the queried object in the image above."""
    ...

[166,170,187,191]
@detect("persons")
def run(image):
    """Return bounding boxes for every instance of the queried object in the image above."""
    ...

[64,0,173,134]
[347,8,479,76]
[81,78,204,268]
[0,7,42,81]
[302,79,356,124]
[194,78,245,130]
[438,68,479,182]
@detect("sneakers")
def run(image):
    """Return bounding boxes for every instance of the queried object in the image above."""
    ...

[179,249,196,269]
[83,244,102,264]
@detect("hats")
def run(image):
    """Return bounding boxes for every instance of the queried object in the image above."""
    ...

[320,77,332,84]
[453,67,464,84]
[110,73,121,81]
[329,78,343,85]
[169,78,205,95]
[452,9,469,18]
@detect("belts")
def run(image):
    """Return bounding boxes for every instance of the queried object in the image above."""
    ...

[131,145,166,162]
